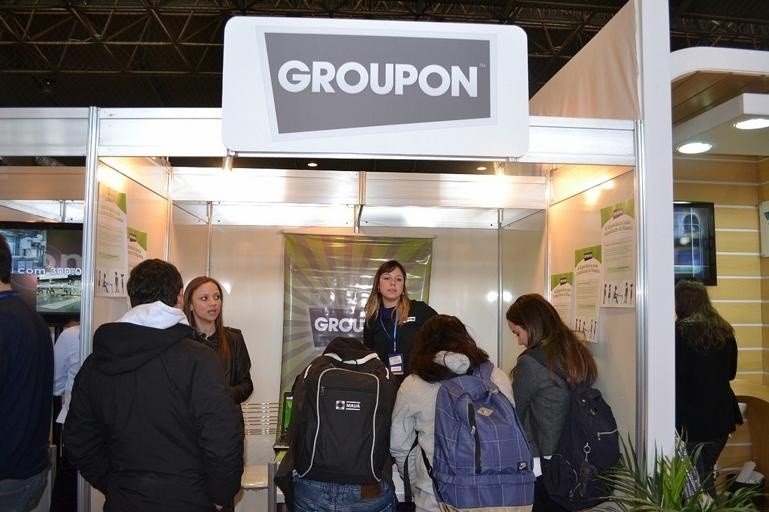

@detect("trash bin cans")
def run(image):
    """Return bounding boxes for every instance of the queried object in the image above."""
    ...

[726,473,765,512]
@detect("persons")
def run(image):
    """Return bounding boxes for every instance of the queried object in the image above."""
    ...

[506,294,598,512]
[362,260,438,386]
[675,277,743,500]
[63,258,245,512]
[0,231,54,511]
[184,276,253,410]
[274,337,398,511]
[54,321,80,512]
[389,314,515,511]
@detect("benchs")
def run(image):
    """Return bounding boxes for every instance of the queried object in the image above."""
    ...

[241,464,269,489]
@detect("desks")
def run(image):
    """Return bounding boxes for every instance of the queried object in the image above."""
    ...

[268,450,407,512]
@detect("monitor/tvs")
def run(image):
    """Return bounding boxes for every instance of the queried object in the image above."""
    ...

[0,221,82,319]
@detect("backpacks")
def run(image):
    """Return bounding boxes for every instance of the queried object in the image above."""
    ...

[291,356,389,485]
[528,351,619,512]
[422,361,536,511]
